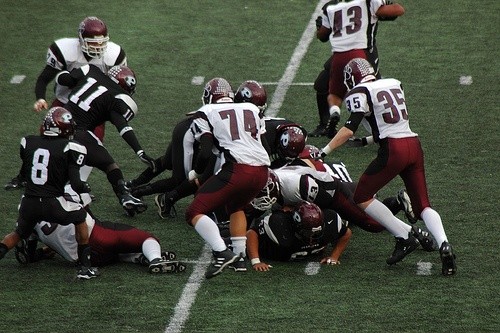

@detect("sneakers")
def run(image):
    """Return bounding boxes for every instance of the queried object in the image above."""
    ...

[412,226,435,251]
[386,229,420,264]
[204,247,240,278]
[154,192,177,220]
[74,267,100,279]
[439,240,457,275]
[119,194,148,213]
[228,252,247,272]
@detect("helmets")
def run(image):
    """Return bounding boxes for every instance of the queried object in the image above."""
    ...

[235,79,267,107]
[299,144,322,161]
[291,203,323,238]
[41,107,72,137]
[276,124,309,158]
[79,15,107,45]
[107,65,136,92]
[345,57,375,88]
[205,76,234,103]
[257,167,281,203]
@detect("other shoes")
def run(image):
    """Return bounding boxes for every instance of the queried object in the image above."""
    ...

[397,188,418,224]
[15,239,30,265]
[325,111,340,138]
[4,176,22,192]
[149,260,186,275]
[124,179,137,191]
[308,126,326,137]
[134,251,177,265]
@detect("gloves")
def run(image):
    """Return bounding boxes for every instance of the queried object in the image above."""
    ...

[138,150,159,173]
[84,181,91,193]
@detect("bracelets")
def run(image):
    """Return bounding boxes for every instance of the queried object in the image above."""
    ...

[322,144,331,155]
[250,258,260,265]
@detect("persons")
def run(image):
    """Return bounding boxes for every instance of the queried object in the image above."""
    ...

[0,0,458,281]
